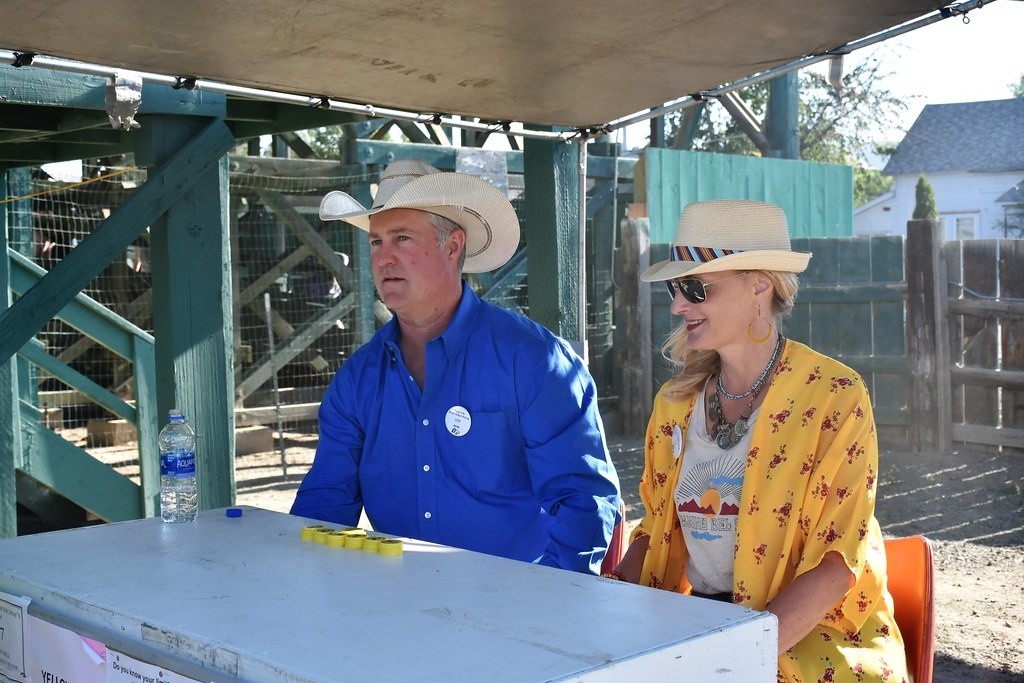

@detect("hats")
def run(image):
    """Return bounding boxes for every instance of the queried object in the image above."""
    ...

[319,160,521,274]
[638,199,813,282]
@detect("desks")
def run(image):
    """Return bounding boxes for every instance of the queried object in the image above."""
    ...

[0,504,778,683]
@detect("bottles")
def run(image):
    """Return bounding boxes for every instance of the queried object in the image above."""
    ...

[158,408,199,523]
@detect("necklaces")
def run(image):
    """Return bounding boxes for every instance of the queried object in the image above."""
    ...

[708,330,786,449]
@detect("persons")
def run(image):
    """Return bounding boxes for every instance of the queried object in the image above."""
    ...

[599,200,909,683]
[287,158,620,577]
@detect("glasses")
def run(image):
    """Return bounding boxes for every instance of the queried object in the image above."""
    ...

[665,271,751,304]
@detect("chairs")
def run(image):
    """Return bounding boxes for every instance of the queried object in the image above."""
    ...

[883,533,939,683]
